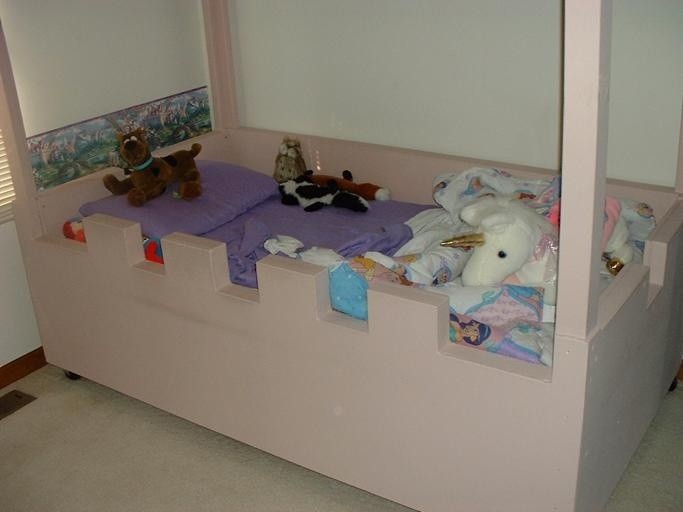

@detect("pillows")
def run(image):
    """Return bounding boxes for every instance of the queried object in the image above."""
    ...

[78,159,279,243]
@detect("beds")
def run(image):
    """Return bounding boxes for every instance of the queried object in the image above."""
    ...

[1,0,683,512]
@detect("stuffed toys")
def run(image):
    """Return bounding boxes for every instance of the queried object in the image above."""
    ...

[278,174,371,214]
[273,135,391,203]
[438,191,635,308]
[103,126,202,208]
